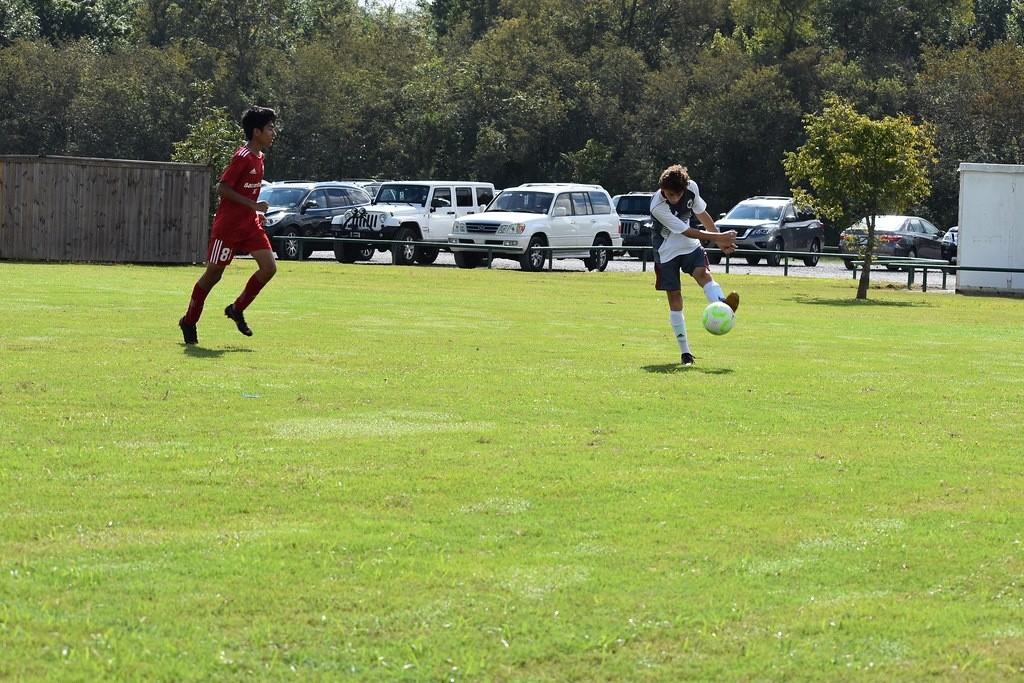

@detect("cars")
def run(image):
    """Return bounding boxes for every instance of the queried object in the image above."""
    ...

[838,215,958,274]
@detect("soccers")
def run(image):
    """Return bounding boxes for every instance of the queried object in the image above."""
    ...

[701,302,736,335]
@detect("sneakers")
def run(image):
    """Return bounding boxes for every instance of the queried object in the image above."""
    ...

[681,353,697,366]
[718,290,740,313]
[224,304,253,337]
[179,316,199,345]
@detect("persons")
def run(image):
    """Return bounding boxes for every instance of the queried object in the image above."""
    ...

[650,165,740,363]
[179,107,277,345]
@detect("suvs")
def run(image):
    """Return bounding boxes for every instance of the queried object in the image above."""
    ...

[330,181,495,264]
[255,181,373,261]
[256,177,654,260]
[448,182,624,273]
[705,195,825,267]
